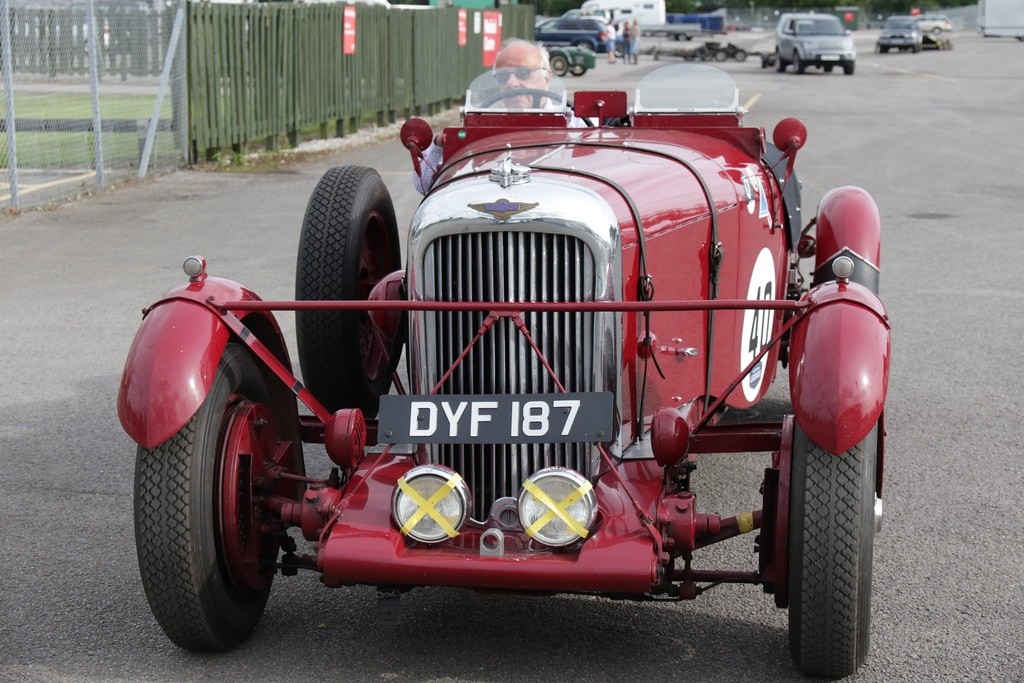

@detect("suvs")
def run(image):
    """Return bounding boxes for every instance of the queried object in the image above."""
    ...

[775,12,857,76]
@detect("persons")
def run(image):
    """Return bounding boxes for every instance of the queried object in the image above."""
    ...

[606,18,640,64]
[413,37,589,196]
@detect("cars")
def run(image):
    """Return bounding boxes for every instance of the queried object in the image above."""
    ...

[115,68,891,680]
[917,13,952,35]
[877,15,921,55]
[560,9,581,19]
[535,18,614,53]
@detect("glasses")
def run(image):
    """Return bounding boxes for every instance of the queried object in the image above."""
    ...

[493,66,551,86]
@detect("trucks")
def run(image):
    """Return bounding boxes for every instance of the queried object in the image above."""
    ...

[579,1,666,37]
